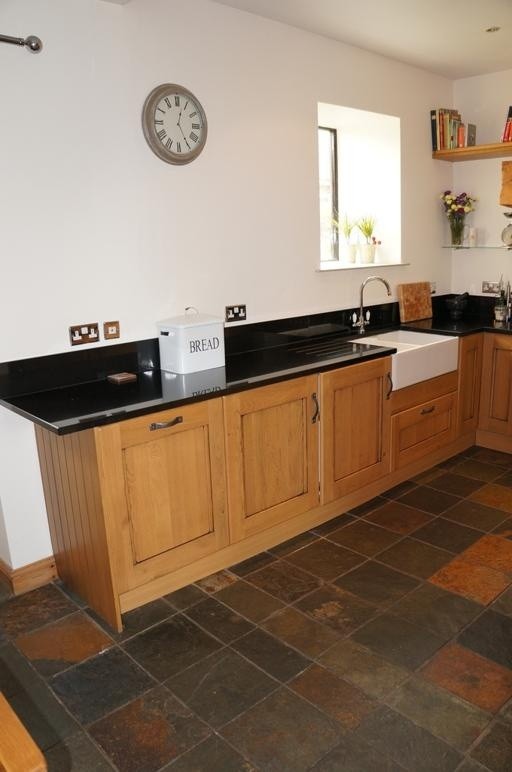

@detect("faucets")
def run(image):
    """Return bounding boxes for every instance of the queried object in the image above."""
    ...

[360,275,391,333]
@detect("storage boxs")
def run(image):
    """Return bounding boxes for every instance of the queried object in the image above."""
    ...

[160,368,227,407]
[155,313,230,374]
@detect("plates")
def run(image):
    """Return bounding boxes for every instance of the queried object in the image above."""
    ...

[501,224,512,246]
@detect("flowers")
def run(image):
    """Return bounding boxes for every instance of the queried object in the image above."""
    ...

[440,190,481,218]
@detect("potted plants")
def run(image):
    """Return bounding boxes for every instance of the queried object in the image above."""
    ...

[356,217,383,265]
[330,212,359,266]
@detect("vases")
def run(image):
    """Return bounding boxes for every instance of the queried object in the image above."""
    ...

[448,215,465,248]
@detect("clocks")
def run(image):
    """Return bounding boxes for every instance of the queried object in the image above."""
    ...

[141,81,212,166]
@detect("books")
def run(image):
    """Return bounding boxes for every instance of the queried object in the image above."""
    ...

[430,105,512,151]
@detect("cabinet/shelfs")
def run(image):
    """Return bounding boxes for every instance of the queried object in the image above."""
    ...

[321,355,394,525]
[389,369,459,488]
[31,369,321,631]
[458,335,482,454]
[479,331,511,452]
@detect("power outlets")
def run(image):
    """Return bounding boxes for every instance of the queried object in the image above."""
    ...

[69,322,101,346]
[224,304,247,323]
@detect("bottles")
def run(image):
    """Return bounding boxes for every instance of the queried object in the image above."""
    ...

[494,290,507,322]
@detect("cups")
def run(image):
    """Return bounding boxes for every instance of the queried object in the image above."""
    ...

[468,227,478,247]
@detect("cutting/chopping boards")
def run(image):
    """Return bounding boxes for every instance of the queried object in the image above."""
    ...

[402,319,433,330]
[397,281,433,323]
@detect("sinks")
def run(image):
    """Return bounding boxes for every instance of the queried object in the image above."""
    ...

[347,330,459,392]
